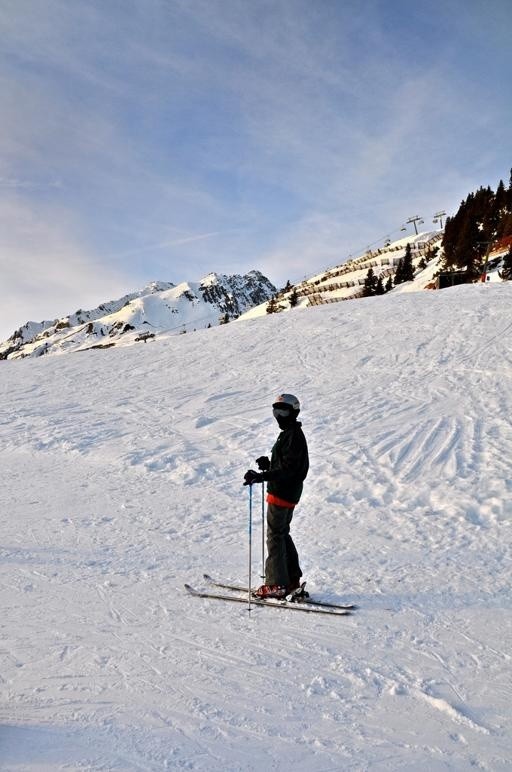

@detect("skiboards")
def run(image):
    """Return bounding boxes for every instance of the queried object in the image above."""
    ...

[184,572,356,616]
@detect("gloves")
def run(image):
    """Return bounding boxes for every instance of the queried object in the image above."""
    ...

[256,456,270,470]
[243,470,265,486]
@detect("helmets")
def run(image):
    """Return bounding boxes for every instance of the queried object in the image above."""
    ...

[271,394,301,412]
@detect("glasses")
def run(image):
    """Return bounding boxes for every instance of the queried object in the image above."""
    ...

[272,408,292,419]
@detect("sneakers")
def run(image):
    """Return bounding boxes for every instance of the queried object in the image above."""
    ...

[252,583,287,599]
[287,582,301,594]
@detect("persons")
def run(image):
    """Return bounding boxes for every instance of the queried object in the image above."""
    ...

[244,393,309,598]
